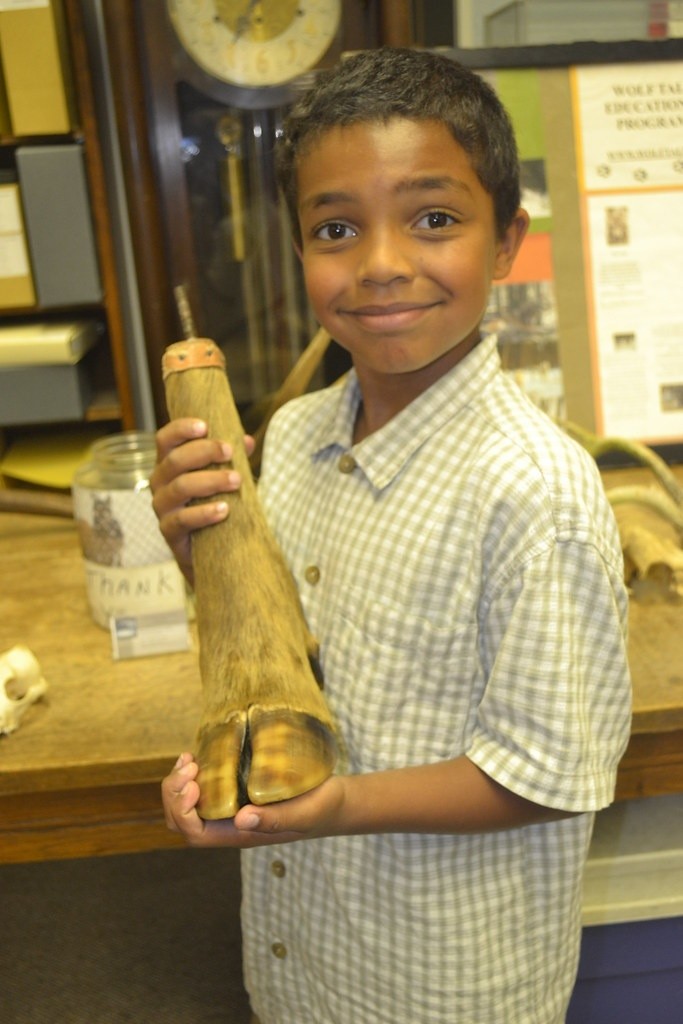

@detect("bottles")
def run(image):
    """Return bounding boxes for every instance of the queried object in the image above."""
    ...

[70,430,186,630]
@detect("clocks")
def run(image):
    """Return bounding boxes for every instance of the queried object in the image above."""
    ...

[165,0,343,111]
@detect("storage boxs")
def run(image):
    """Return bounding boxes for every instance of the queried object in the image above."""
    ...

[563,795,683,1024]
[0,0,102,426]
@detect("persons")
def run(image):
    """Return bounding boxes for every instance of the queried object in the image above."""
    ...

[148,46,633,1024]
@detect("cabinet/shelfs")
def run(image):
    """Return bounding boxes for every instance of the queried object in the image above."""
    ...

[0,0,176,532]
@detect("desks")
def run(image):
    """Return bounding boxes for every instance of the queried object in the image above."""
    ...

[0,464,683,860]
